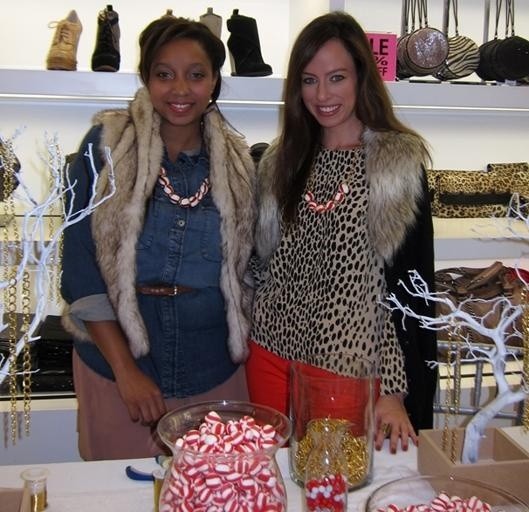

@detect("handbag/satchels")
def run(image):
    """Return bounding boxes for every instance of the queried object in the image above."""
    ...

[394,0,450,78]
[429,0,482,81]
[421,161,529,223]
[431,260,528,366]
[474,0,529,82]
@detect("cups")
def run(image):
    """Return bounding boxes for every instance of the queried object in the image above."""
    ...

[290,355,376,487]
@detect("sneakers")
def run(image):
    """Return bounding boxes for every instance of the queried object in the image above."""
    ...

[90,4,121,72]
[198,5,223,44]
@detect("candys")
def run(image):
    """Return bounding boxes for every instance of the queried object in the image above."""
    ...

[305,472,347,512]
[378,493,492,512]
[159,411,286,512]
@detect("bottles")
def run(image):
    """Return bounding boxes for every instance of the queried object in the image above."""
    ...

[22,468,46,508]
[307,420,348,508]
[159,399,288,512]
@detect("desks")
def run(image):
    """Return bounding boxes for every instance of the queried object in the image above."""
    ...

[1,426,528,512]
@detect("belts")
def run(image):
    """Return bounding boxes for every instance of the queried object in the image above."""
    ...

[133,283,197,298]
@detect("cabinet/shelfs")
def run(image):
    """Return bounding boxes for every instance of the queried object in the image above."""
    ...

[1,70,529,466]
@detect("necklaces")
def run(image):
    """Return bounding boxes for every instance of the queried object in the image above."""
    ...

[298,138,362,215]
[153,164,210,207]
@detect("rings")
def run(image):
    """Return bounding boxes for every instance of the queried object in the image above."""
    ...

[379,422,391,437]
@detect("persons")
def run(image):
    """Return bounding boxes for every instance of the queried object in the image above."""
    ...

[240,9,441,457]
[48,14,263,463]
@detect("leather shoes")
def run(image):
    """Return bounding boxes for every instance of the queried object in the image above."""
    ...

[45,8,84,72]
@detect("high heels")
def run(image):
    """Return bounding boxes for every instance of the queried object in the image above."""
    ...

[225,7,274,78]
